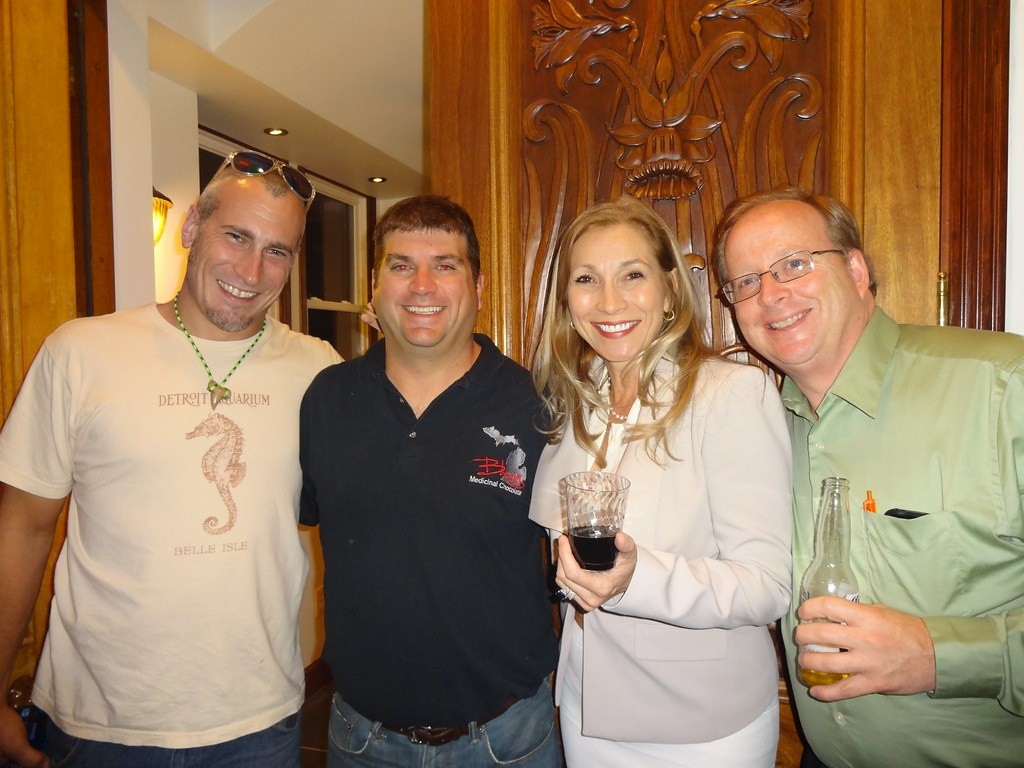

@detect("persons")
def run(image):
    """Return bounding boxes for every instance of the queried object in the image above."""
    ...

[1,151,1024,768]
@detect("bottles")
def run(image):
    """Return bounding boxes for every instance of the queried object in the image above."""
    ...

[796,477,860,687]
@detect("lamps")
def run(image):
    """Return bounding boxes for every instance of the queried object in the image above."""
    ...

[153,187,173,246]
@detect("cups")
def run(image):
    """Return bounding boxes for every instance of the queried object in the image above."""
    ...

[562,471,632,571]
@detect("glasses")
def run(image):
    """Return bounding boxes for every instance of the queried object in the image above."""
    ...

[719,250,847,304]
[211,149,316,214]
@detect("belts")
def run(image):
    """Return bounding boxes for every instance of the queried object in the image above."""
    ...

[345,695,522,747]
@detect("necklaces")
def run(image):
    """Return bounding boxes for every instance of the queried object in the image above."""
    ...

[607,377,630,420]
[171,290,268,410]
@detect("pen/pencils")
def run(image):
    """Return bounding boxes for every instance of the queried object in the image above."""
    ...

[864,491,876,515]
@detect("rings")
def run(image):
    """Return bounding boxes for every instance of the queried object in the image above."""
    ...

[557,587,575,601]
